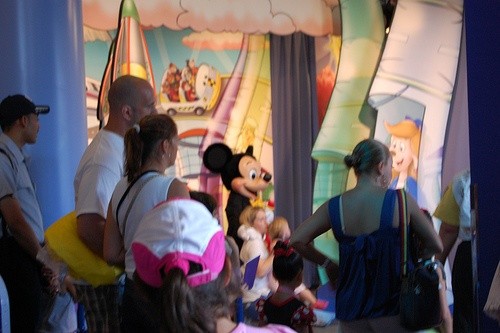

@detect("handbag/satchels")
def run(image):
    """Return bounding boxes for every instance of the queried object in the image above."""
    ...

[399,262,441,332]
[45,210,125,288]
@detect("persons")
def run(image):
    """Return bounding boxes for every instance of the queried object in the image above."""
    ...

[265,217,330,310]
[74,74,160,333]
[432,166,479,333]
[408,209,453,333]
[188,190,249,321]
[127,198,300,333]
[237,203,335,333]
[0,93,58,333]
[288,137,445,333]
[102,114,191,333]
[251,241,318,333]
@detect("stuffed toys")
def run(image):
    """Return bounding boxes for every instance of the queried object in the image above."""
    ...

[202,143,272,266]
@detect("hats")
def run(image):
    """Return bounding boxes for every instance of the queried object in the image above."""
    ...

[131,199,226,290]
[0,94,51,132]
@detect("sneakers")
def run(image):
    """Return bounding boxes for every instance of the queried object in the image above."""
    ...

[313,299,329,310]
